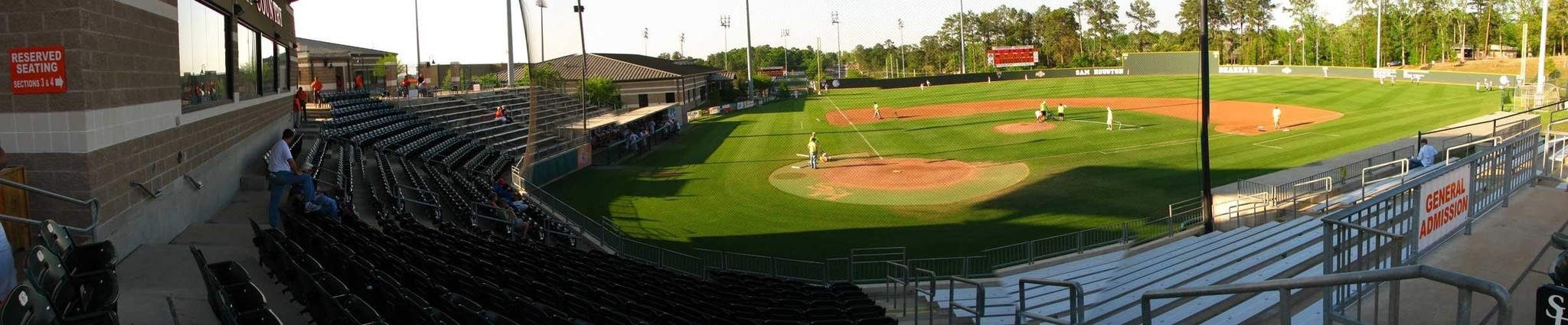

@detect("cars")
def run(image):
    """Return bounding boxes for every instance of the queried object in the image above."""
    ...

[1386,60,1402,67]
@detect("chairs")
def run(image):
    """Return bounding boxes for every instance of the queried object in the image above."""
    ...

[247,210,899,325]
[262,128,577,248]
[189,244,283,324]
[1,219,120,324]
[319,89,515,181]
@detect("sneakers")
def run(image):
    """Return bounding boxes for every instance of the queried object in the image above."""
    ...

[811,166,819,169]
[1107,127,1112,130]
[304,202,321,213]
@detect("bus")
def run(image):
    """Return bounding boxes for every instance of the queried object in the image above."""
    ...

[1269,60,1284,65]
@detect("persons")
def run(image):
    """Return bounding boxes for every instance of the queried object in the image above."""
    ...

[1379,73,1422,88]
[496,104,514,124]
[401,76,411,98]
[825,83,829,90]
[1322,67,1329,79]
[1272,107,1281,129]
[268,128,337,227]
[988,76,990,83]
[921,83,925,91]
[1476,78,1494,93]
[487,177,530,239]
[294,93,301,128]
[594,118,681,153]
[311,76,323,106]
[1058,104,1064,121]
[354,72,364,90]
[873,102,881,119]
[1106,107,1113,130]
[1025,74,1028,80]
[416,73,426,97]
[298,87,308,121]
[807,131,820,169]
[927,80,931,87]
[1036,101,1048,123]
[1409,138,1438,168]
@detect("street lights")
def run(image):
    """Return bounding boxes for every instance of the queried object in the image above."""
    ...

[201,64,206,78]
[781,28,790,78]
[830,11,841,78]
[643,28,648,56]
[720,15,730,70]
[536,0,546,62]
[680,33,685,57]
[897,18,905,78]
[357,54,364,77]
[429,55,435,65]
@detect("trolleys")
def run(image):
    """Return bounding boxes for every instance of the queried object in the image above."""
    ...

[1046,108,1056,121]
